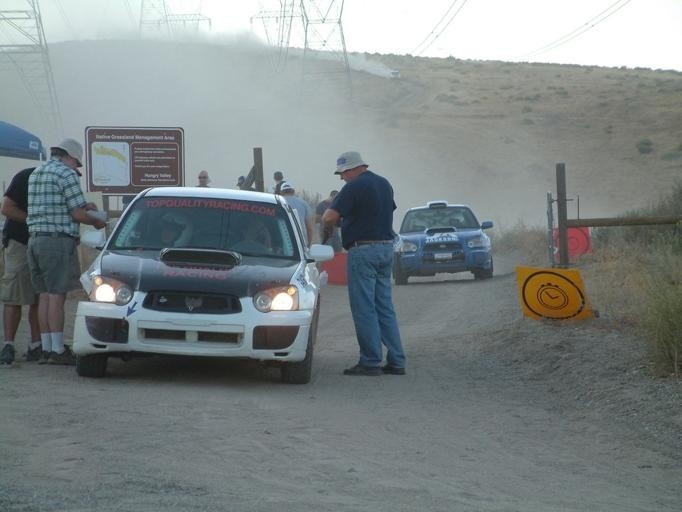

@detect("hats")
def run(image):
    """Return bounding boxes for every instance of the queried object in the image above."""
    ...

[333,152,368,175]
[50,139,84,166]
[276,181,296,196]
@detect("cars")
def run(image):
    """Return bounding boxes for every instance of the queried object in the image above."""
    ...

[392,199,500,282]
[72,183,334,388]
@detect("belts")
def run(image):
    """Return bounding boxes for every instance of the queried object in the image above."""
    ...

[27,232,74,238]
[348,240,394,248]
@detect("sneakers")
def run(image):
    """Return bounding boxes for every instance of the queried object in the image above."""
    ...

[342,365,383,377]
[381,364,406,375]
[39,344,77,363]
[0,344,15,365]
[27,344,41,360]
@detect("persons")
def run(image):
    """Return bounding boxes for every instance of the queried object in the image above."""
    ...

[316,191,342,252]
[280,183,312,247]
[125,211,192,248]
[322,152,406,375]
[196,171,208,186]
[238,176,256,191]
[0,168,42,364]
[267,172,283,194]
[26,140,107,366]
